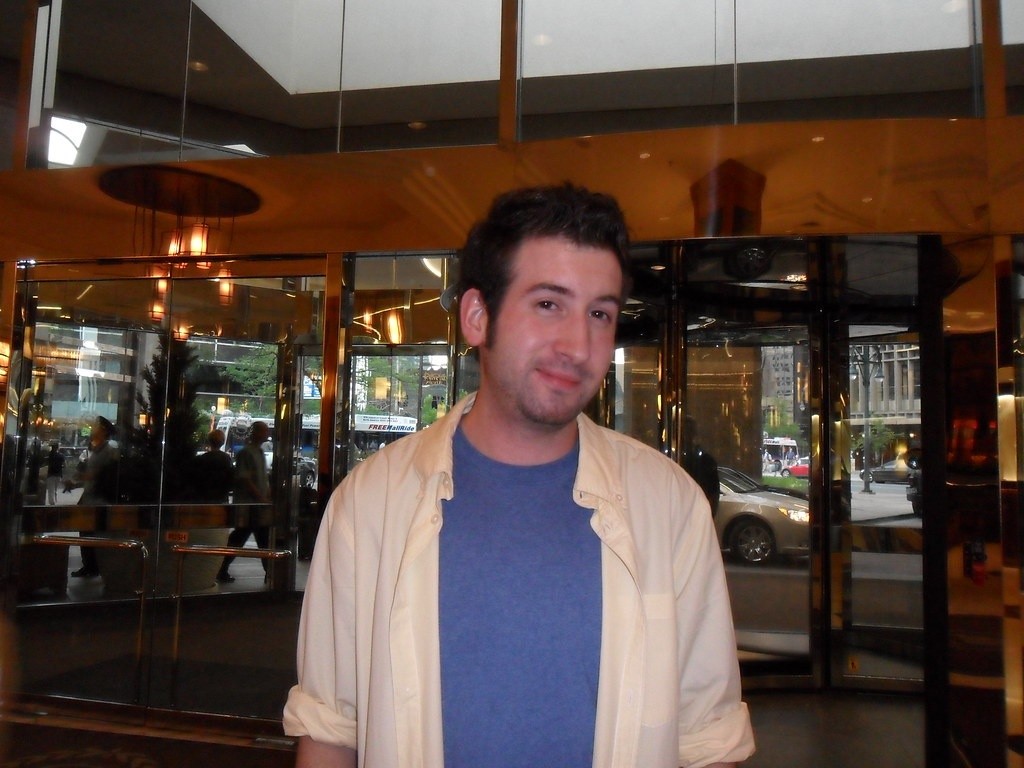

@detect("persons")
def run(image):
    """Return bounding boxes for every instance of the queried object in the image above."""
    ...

[62,416,119,577]
[47,443,65,505]
[762,449,772,473]
[785,447,796,466]
[684,416,720,518]
[217,421,272,584]
[283,184,755,768]
[195,429,236,504]
[370,438,386,453]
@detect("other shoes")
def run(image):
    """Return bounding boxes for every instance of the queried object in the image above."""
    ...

[216,571,235,582]
[71,566,95,577]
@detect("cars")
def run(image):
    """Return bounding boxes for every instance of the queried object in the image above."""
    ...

[710,466,810,567]
[781,456,810,478]
[261,440,317,491]
[860,459,921,488]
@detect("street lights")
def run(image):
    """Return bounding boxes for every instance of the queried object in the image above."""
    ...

[850,356,886,496]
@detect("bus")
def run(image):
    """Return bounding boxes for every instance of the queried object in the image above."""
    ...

[764,436,800,473]
[215,411,429,497]
